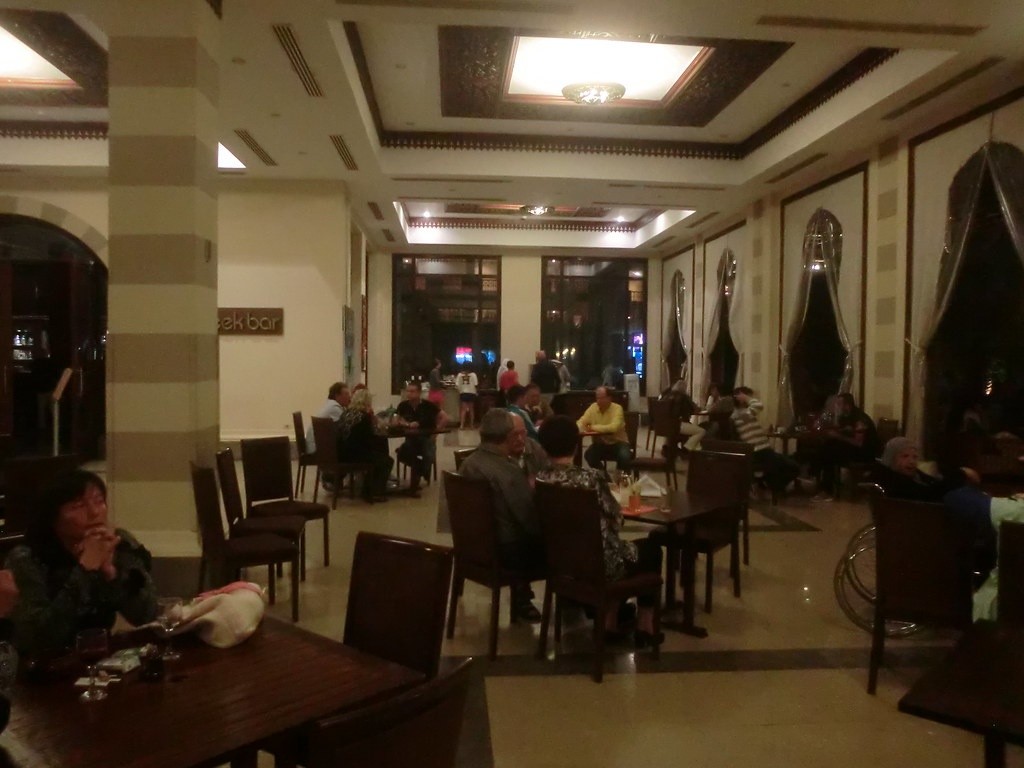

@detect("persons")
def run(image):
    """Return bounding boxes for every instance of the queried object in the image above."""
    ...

[530,350,562,410]
[652,374,808,499]
[0,570,19,736]
[873,436,1024,618]
[500,360,520,406]
[575,385,634,474]
[454,404,552,618]
[806,393,882,502]
[395,381,447,484]
[454,363,478,429]
[505,382,554,439]
[2,457,160,652]
[304,382,394,503]
[429,358,446,409]
[533,412,666,649]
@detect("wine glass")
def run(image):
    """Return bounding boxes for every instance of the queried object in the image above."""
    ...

[76,627,110,702]
[155,597,184,661]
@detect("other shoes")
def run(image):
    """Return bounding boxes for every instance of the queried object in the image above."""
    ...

[809,491,834,502]
[515,599,541,620]
[585,602,636,620]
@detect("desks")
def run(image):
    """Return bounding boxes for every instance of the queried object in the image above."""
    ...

[376,427,452,492]
[476,389,500,423]
[767,432,810,497]
[0,615,428,768]
[898,618,1024,768]
[549,389,629,421]
[620,491,721,638]
[691,410,708,416]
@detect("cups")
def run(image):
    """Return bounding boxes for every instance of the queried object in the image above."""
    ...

[659,486,673,513]
[629,495,641,512]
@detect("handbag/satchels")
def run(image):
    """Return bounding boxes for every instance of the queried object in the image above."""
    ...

[132,582,269,648]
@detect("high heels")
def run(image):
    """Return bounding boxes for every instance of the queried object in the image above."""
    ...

[633,627,665,648]
[603,628,624,640]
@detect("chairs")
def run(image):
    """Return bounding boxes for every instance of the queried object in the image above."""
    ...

[240,436,330,566]
[868,495,980,696]
[442,470,545,661]
[852,419,898,473]
[651,451,747,614]
[700,438,756,565]
[634,414,678,491]
[308,655,494,768]
[601,412,639,470]
[343,530,455,680]
[651,404,676,458]
[646,397,657,449]
[215,447,306,580]
[311,417,374,511]
[292,412,317,498]
[189,460,299,622]
[395,408,441,481]
[0,568,19,618]
[533,476,665,682]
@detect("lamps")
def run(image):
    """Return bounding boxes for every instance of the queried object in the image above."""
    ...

[562,83,626,106]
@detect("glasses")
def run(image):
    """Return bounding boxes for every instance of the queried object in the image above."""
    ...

[511,429,528,439]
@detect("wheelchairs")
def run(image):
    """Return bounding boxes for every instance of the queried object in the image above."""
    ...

[834,482,932,640]
